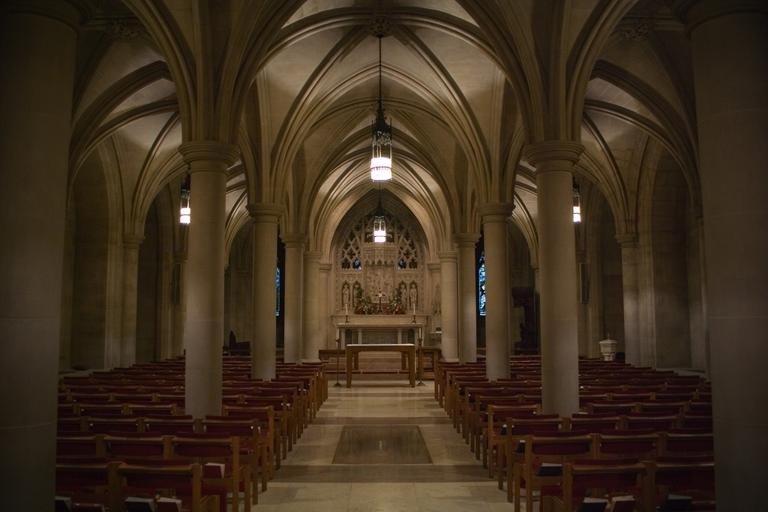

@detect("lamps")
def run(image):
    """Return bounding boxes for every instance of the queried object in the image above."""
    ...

[571,191,582,224]
[373,216,387,243]
[370,126,393,183]
[179,190,192,225]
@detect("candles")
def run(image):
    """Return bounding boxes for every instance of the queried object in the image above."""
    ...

[345,303,348,314]
[413,302,416,314]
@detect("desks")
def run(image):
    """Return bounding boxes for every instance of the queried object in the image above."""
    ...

[346,342,416,389]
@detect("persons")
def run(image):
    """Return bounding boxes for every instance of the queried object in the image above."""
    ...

[399,283,407,309]
[353,284,362,307]
[342,283,350,310]
[409,283,417,308]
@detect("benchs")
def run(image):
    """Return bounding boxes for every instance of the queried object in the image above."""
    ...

[58,352,328,511]
[433,352,714,510]
